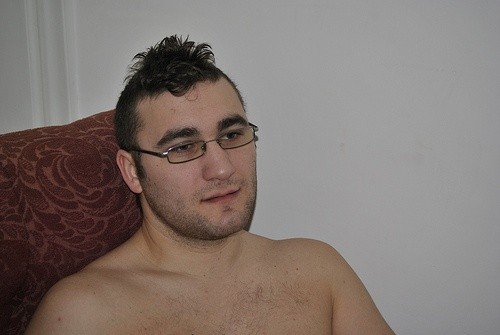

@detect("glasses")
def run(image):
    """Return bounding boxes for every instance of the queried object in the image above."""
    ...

[127,122,259,164]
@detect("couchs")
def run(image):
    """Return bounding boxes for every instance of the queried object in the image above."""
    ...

[0,108,144,335]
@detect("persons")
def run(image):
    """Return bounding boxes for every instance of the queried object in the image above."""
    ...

[24,34,396,335]
